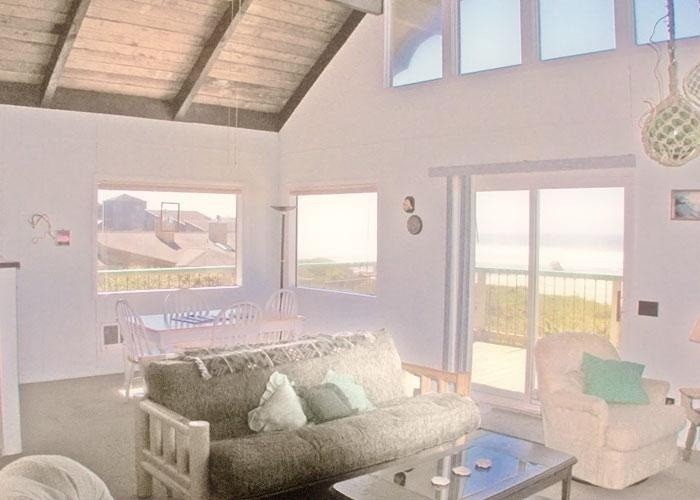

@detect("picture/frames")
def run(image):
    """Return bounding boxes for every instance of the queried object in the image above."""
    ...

[670,189,700,222]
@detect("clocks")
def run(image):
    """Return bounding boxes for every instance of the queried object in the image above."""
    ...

[407,215,422,235]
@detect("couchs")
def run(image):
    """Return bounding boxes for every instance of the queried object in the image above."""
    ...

[133,326,480,500]
[533,332,686,492]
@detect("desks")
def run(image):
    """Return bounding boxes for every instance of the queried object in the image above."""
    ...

[125,309,305,353]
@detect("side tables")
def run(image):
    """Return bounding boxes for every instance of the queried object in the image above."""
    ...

[680,388,700,461]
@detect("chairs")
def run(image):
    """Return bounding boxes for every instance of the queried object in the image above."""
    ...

[163,290,207,311]
[115,299,157,403]
[262,289,297,344]
[213,302,262,350]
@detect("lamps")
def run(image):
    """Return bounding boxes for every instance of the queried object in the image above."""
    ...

[689,314,700,344]
[270,205,297,341]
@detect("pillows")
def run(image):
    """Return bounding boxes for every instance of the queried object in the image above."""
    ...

[295,371,375,424]
[581,352,649,405]
[247,371,307,433]
